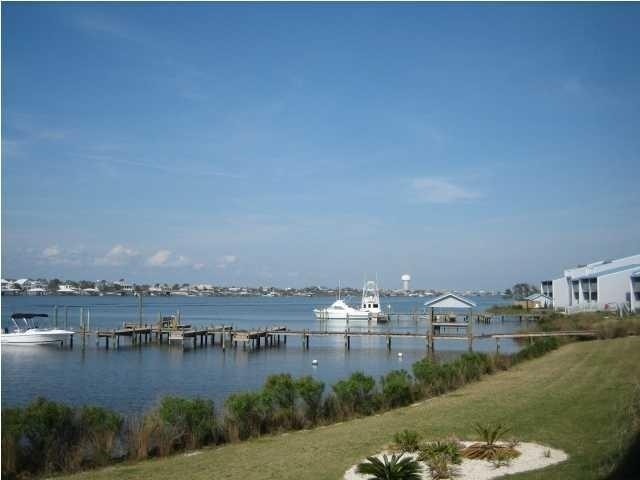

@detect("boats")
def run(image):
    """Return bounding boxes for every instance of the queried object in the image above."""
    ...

[1,313,76,346]
[311,270,383,319]
[124,315,190,328]
